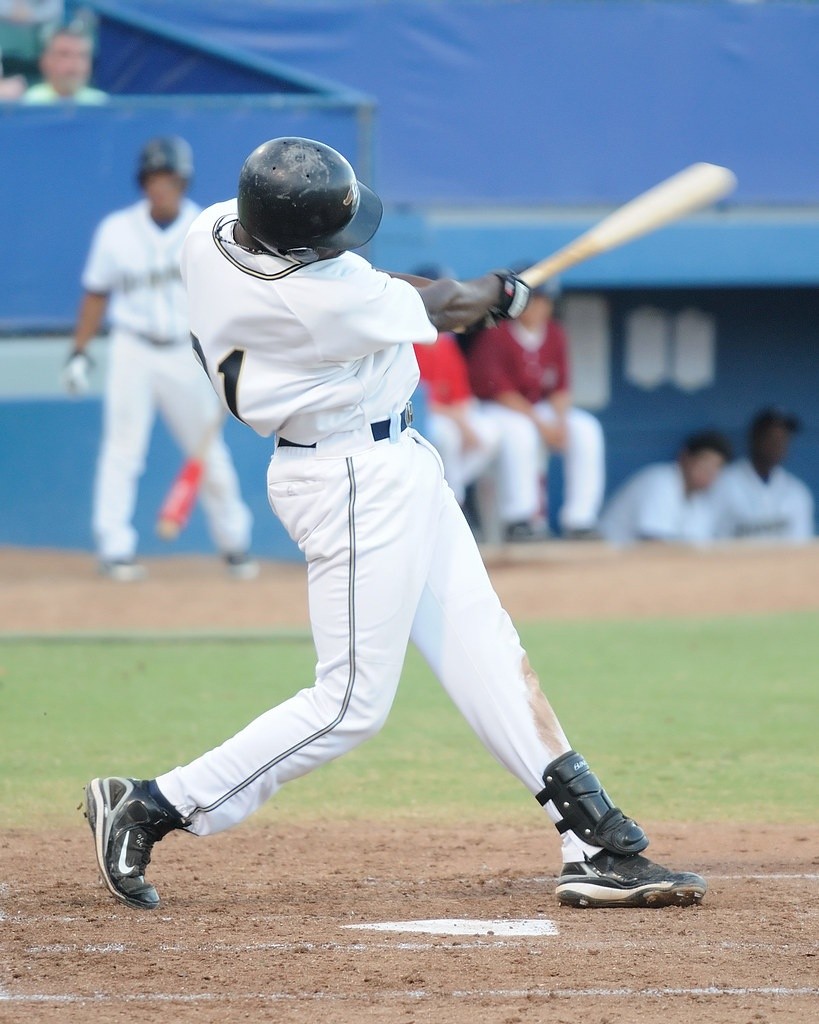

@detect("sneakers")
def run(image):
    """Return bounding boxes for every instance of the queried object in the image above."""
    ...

[87,774,176,905]
[559,846,708,904]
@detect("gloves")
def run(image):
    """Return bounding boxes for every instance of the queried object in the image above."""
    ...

[492,271,531,320]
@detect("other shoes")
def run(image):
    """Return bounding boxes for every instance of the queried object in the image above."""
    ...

[220,547,258,579]
[100,560,141,581]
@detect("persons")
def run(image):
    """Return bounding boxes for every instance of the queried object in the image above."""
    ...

[0,0,109,104]
[76,136,706,910]
[403,258,815,549]
[59,137,263,584]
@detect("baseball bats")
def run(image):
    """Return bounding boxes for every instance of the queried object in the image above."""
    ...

[516,162,737,289]
[154,406,232,540]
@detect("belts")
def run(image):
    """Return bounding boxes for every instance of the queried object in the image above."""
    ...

[276,404,412,450]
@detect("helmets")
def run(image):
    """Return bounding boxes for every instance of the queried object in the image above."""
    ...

[750,401,804,430]
[510,255,562,296]
[237,136,383,258]
[137,134,194,180]
[679,428,737,460]
[405,255,460,282]
